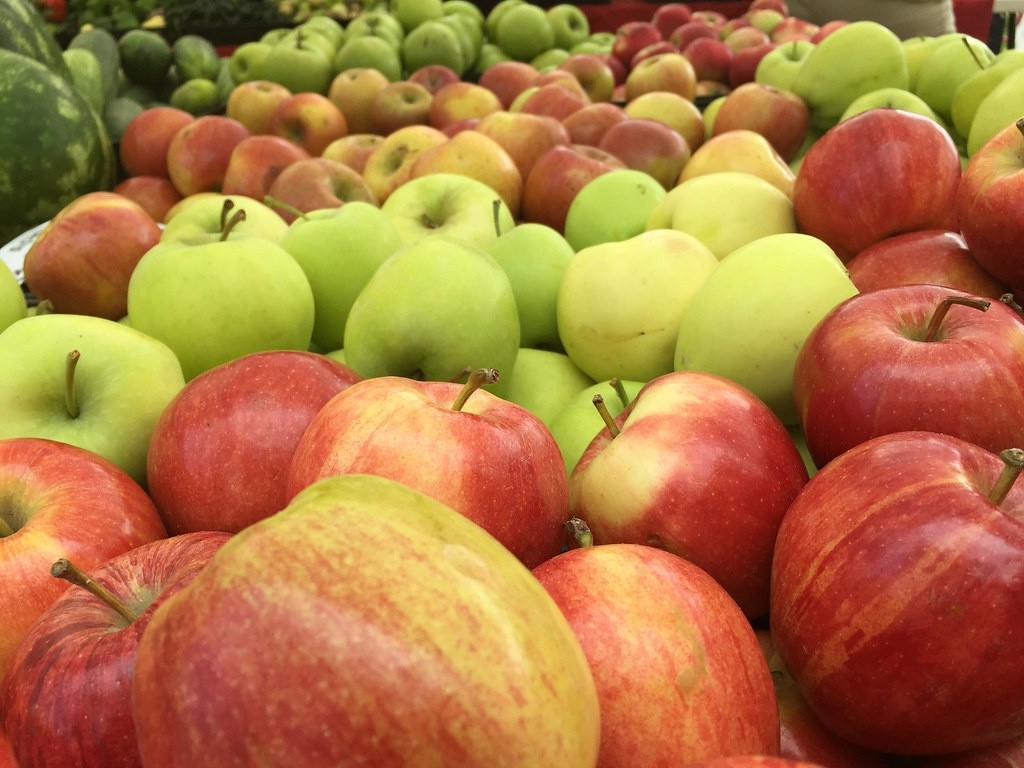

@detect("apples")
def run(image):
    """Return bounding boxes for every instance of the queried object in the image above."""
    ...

[0,0,1024,768]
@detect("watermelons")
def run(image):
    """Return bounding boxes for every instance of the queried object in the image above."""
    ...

[0,49,119,248]
[0,0,77,88]
